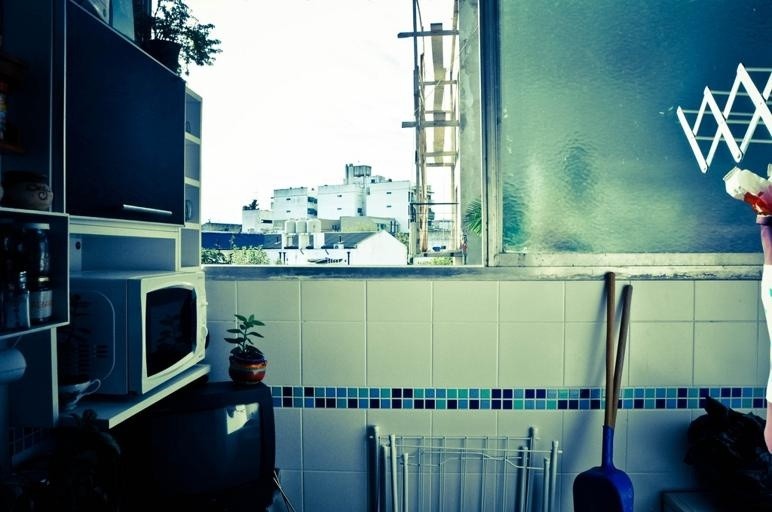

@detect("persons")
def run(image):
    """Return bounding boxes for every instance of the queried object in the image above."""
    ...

[755,215,772,456]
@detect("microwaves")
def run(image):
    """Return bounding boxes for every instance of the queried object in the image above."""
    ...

[57,266,210,399]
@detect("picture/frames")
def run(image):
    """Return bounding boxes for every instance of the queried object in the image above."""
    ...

[76,0,115,28]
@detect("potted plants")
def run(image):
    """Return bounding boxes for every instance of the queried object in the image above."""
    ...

[141,1,224,79]
[223,310,270,388]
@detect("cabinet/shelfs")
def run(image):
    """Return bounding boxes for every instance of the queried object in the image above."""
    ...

[0,83,213,470]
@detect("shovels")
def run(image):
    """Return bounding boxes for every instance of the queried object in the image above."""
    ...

[573,272,634,512]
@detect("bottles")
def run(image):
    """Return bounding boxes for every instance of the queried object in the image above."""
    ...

[1,217,56,333]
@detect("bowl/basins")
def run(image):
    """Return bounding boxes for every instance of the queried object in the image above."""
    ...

[58,373,101,413]
